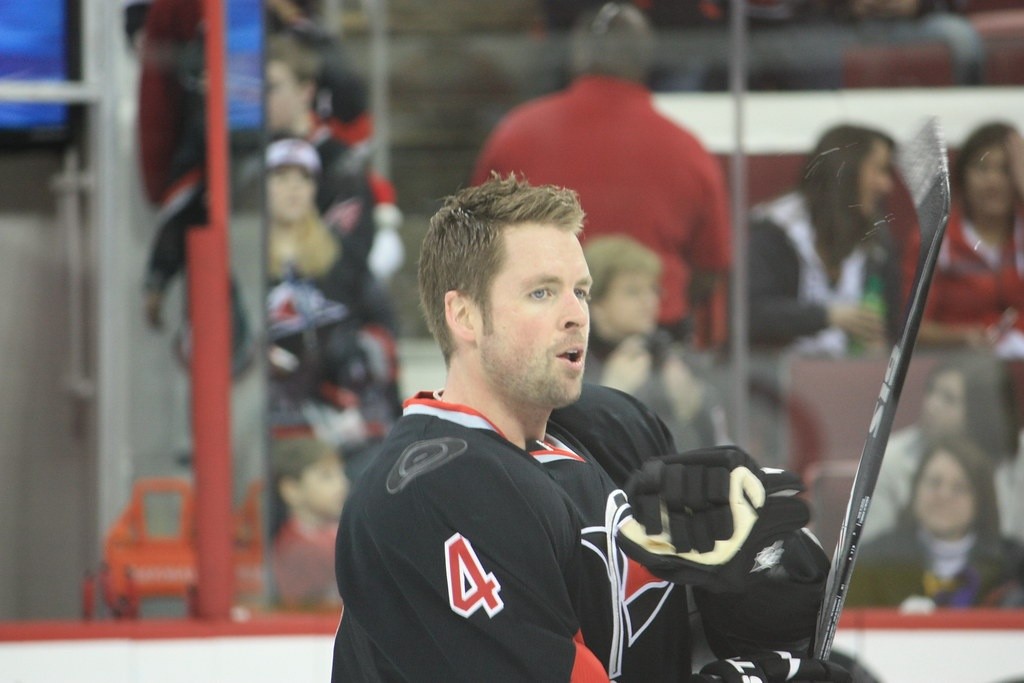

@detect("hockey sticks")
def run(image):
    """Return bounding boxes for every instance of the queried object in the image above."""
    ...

[809,110,958,666]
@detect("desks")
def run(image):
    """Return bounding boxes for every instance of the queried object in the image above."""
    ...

[837,609,1024,683]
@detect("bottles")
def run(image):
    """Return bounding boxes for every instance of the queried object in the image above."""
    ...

[848,271,885,354]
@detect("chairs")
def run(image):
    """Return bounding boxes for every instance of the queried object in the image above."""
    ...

[102,477,206,622]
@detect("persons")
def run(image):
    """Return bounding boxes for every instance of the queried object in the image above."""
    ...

[119,0,401,531]
[663,0,985,91]
[551,386,880,683]
[469,4,731,353]
[842,436,1024,613]
[270,441,357,607]
[744,122,907,399]
[896,124,1023,361]
[330,174,853,683]
[863,345,1024,538]
[573,235,736,451]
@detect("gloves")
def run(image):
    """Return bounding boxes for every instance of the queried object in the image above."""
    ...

[613,446,812,597]
[693,649,854,683]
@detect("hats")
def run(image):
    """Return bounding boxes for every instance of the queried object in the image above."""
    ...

[266,282,344,340]
[264,136,321,176]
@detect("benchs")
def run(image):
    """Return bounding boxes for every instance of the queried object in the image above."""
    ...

[328,0,1024,514]
